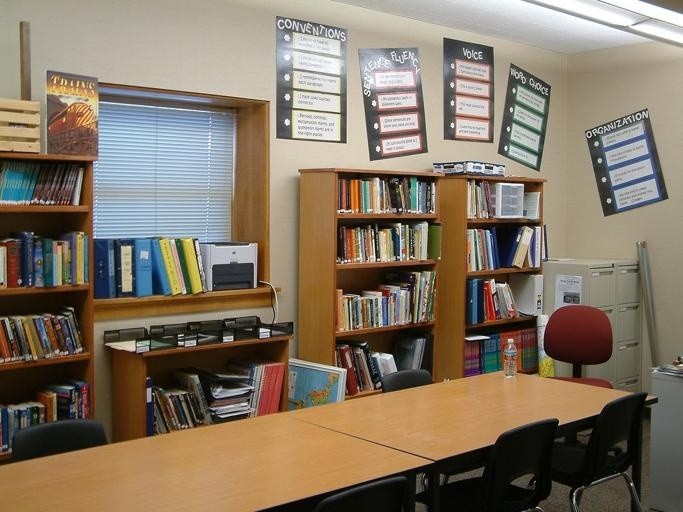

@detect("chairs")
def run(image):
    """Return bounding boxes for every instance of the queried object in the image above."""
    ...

[551,386,649,512]
[543,301,617,392]
[416,418,561,512]
[320,476,411,511]
[380,369,434,392]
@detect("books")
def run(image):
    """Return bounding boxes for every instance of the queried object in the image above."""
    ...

[0,158,91,455]
[338,271,437,330]
[338,334,433,395]
[337,221,443,263]
[93,237,208,298]
[44,68,100,159]
[339,177,442,213]
[145,356,286,437]
[656,368,683,378]
[467,178,541,376]
[659,364,682,372]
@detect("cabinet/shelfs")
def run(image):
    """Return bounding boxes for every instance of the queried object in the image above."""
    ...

[591,268,643,394]
[104,329,297,434]
[1,153,94,462]
[458,178,545,383]
[303,168,445,382]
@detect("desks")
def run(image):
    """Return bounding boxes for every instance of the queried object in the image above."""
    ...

[1,370,658,511]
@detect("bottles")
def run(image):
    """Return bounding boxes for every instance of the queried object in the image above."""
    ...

[503,338,518,379]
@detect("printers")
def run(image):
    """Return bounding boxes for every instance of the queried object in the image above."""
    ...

[200,241,258,292]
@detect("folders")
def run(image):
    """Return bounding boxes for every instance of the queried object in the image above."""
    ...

[500,226,542,268]
[466,278,485,324]
